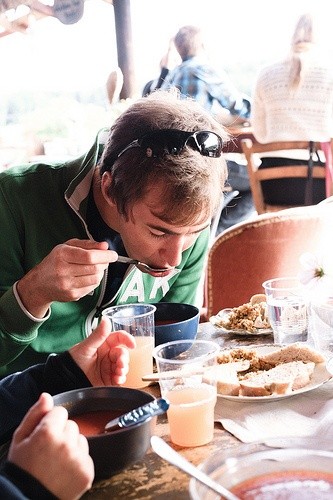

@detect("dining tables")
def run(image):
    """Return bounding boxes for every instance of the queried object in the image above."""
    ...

[83,320,333,500]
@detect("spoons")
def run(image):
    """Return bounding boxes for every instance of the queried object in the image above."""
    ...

[118,254,174,272]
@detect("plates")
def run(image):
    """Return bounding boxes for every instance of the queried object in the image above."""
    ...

[182,342,333,403]
[209,309,273,335]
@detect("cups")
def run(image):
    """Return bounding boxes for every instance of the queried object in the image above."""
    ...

[101,304,156,389]
[262,277,313,342]
[152,339,219,448]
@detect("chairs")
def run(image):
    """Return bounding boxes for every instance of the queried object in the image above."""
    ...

[242,139,333,217]
[204,196,333,321]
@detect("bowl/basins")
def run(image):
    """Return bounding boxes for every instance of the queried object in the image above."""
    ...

[112,302,200,362]
[313,298,333,328]
[51,385,158,479]
[188,436,333,500]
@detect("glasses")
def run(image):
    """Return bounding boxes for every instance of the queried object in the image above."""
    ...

[100,130,226,176]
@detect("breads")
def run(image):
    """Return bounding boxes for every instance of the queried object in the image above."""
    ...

[249,294,267,306]
[259,342,324,365]
[241,361,315,396]
[202,360,251,396]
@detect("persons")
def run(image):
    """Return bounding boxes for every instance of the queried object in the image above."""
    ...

[154,24,253,192]
[0,315,136,500]
[251,15,333,205]
[0,92,229,380]
